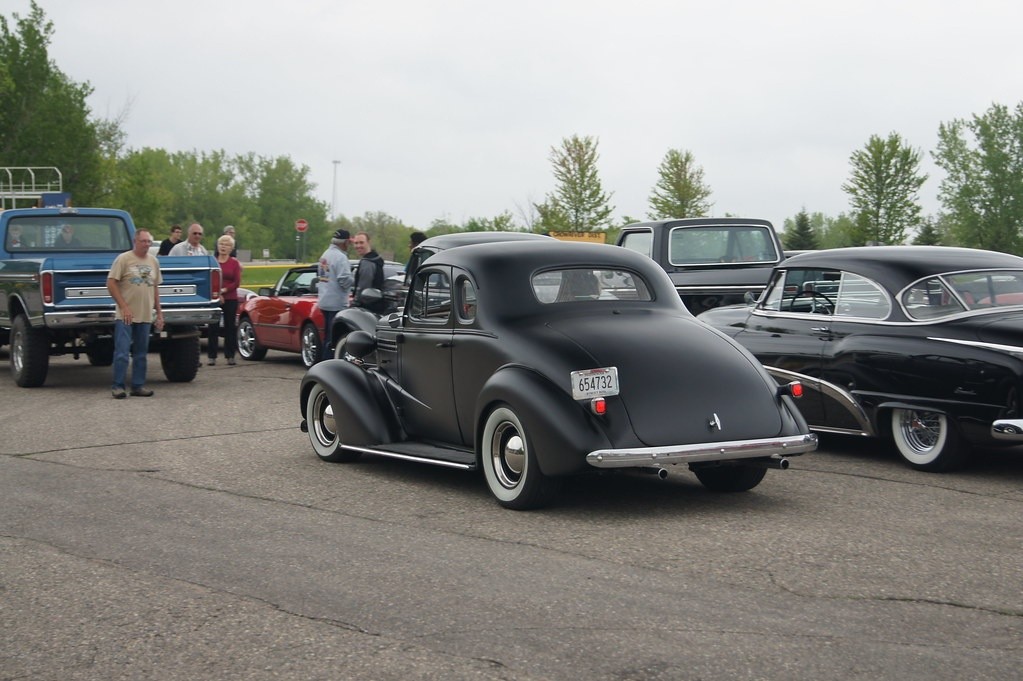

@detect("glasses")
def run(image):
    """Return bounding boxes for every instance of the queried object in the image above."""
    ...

[137,239,152,246]
[193,232,202,236]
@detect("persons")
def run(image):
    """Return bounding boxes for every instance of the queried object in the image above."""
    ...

[354,232,385,297]
[8,224,27,247]
[319,229,355,361]
[54,224,82,248]
[107,229,165,398]
[207,235,242,365]
[213,226,238,259]
[169,224,208,256]
[155,225,183,255]
[405,232,428,274]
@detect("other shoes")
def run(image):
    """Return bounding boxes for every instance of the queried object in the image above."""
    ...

[207,358,215,365]
[227,358,236,365]
[129,387,153,396]
[112,387,126,399]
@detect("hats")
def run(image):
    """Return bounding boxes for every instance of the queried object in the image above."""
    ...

[62,225,73,233]
[333,229,355,243]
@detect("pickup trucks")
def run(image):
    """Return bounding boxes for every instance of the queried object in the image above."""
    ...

[0,205,223,388]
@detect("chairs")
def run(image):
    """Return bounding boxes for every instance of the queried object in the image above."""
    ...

[568,273,601,300]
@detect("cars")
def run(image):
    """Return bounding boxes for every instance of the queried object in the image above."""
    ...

[235,220,819,368]
[697,245,1023,472]
[300,219,818,512]
